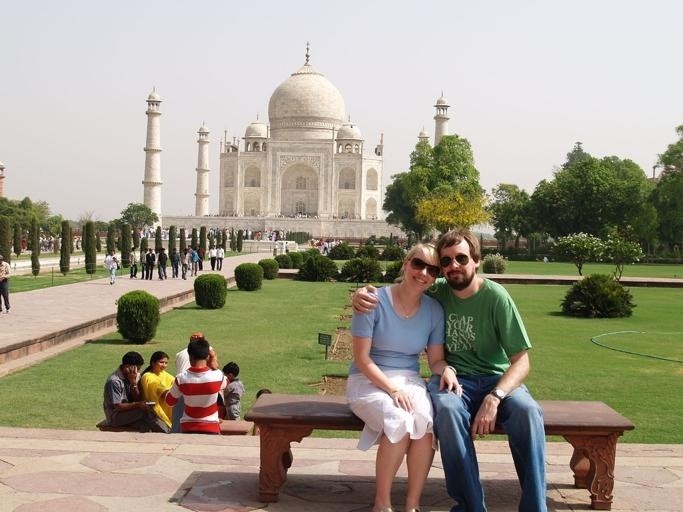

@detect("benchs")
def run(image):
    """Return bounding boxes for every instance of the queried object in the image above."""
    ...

[95,421,255,436]
[244,393,635,511]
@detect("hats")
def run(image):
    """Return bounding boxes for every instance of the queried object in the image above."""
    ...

[0,255,3,260]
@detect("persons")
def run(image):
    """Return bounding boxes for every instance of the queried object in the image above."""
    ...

[285,242,289,253]
[169,248,179,278]
[181,248,190,280]
[310,238,342,255]
[140,248,149,279]
[129,247,138,278]
[209,227,279,242]
[158,248,167,279]
[252,388,273,435]
[222,363,245,419]
[146,248,155,280]
[188,245,193,270]
[208,245,217,271]
[139,351,176,428]
[347,244,463,512]
[217,244,224,271]
[197,246,206,270]
[102,351,170,433]
[39,235,78,252]
[156,249,165,277]
[164,338,229,435]
[172,333,216,433]
[0,255,12,313]
[104,250,119,285]
[190,247,200,277]
[351,228,548,512]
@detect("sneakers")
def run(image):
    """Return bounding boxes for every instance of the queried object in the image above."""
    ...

[110,282,113,285]
[372,508,394,512]
[0,310,2,312]
[7,307,10,313]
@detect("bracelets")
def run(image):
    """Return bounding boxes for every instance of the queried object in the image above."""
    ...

[442,365,456,375]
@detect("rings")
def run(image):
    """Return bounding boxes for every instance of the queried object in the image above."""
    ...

[459,385,463,387]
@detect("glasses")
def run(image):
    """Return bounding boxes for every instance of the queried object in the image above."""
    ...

[439,255,470,266]
[410,257,441,278]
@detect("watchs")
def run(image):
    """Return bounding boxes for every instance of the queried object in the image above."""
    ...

[492,387,506,400]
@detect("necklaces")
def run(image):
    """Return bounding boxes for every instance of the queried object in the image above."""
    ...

[397,291,419,320]
